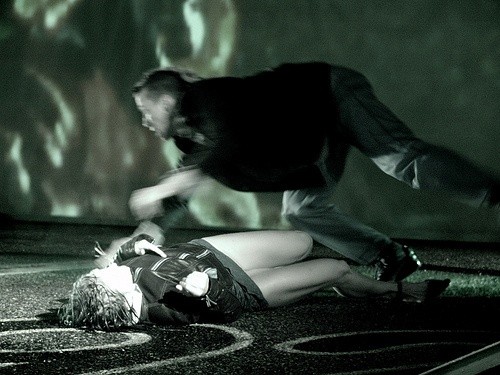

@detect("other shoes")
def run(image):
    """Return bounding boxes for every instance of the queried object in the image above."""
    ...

[472,179,500,210]
[369,240,423,283]
[387,277,450,305]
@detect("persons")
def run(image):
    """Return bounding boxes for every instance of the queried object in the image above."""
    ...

[58,229,452,332]
[130,62,498,284]
